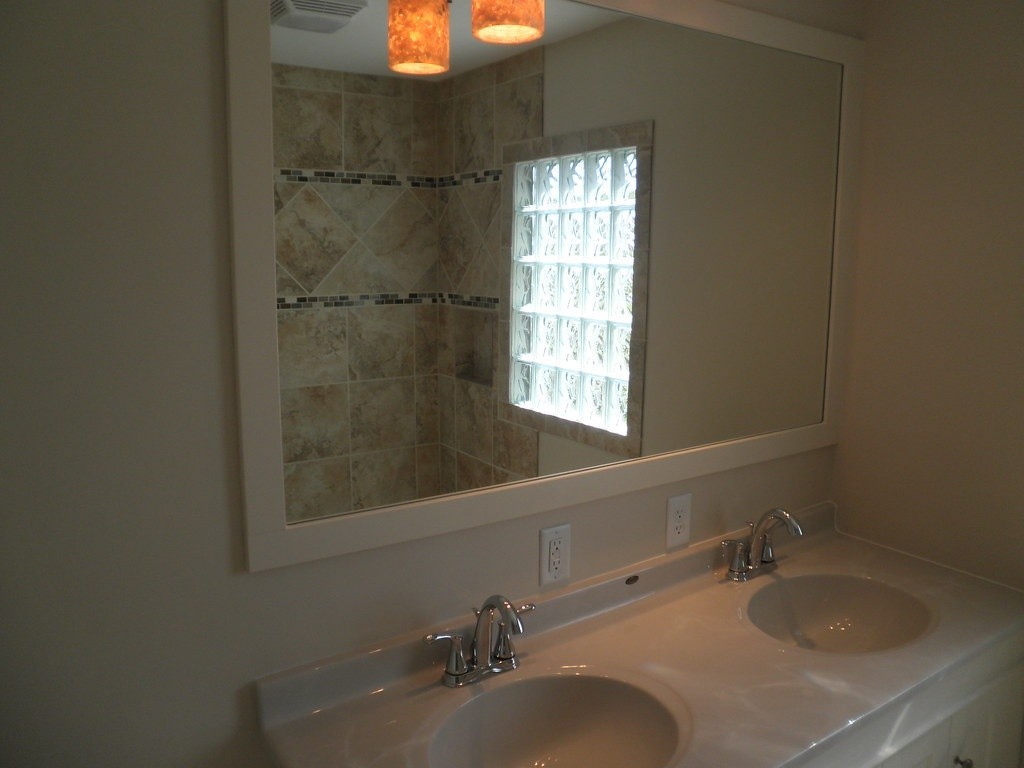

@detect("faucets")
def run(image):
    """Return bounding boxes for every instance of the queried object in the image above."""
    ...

[737,506,808,582]
[465,592,528,674]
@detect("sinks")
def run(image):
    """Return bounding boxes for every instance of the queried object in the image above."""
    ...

[403,657,695,768]
[741,567,942,671]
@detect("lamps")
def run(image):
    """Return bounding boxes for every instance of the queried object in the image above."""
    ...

[387,0,451,76]
[470,2,545,45]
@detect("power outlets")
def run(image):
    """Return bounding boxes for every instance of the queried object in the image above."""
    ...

[538,523,573,586]
[666,491,692,552]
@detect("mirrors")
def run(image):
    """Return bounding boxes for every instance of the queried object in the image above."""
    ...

[222,0,874,576]
